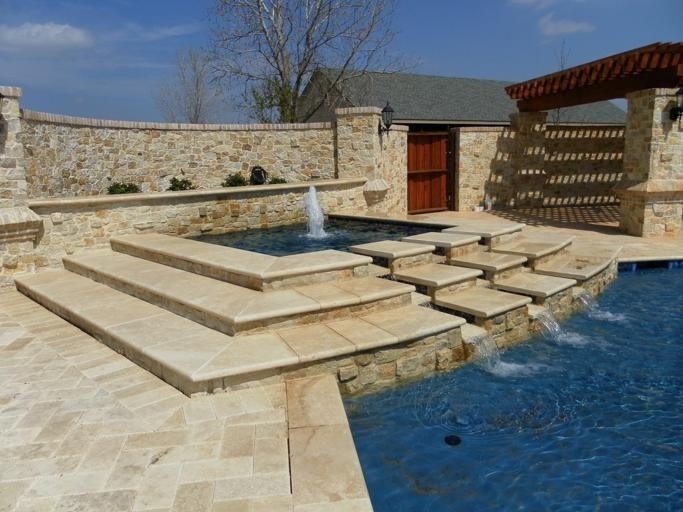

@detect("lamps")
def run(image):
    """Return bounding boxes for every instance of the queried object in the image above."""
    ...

[377,100,393,133]
